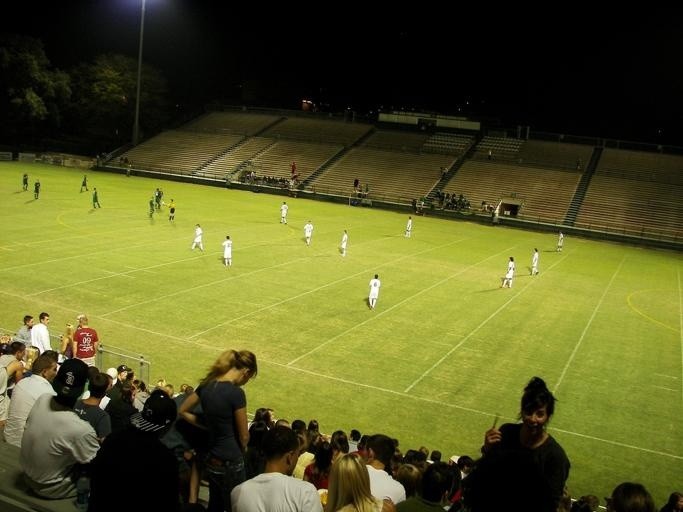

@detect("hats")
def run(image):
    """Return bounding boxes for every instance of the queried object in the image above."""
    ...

[131,394,177,433]
[52,358,88,398]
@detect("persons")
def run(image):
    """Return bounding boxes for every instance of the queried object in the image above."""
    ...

[439,167,449,181]
[154,188,162,208]
[492,148,497,161]
[167,199,175,221]
[92,188,101,209]
[411,189,494,215]
[303,221,313,247]
[500,257,513,289]
[279,202,288,224]
[1,312,683,510]
[364,184,368,196]
[580,158,584,172]
[148,196,154,218]
[124,156,128,168]
[126,163,130,177]
[353,176,358,191]
[340,230,348,256]
[404,215,411,238]
[192,224,203,252]
[118,155,124,168]
[23,171,28,190]
[366,274,380,311]
[221,236,232,267]
[224,161,300,190]
[33,179,40,199]
[556,230,563,253]
[575,158,579,171]
[79,174,88,192]
[357,184,362,198]
[487,148,492,161]
[530,247,538,276]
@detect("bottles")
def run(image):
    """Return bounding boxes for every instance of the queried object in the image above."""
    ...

[58,334,63,344]
[316,487,329,505]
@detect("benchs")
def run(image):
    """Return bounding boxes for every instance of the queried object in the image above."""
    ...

[0,438,87,510]
[98,110,682,251]
